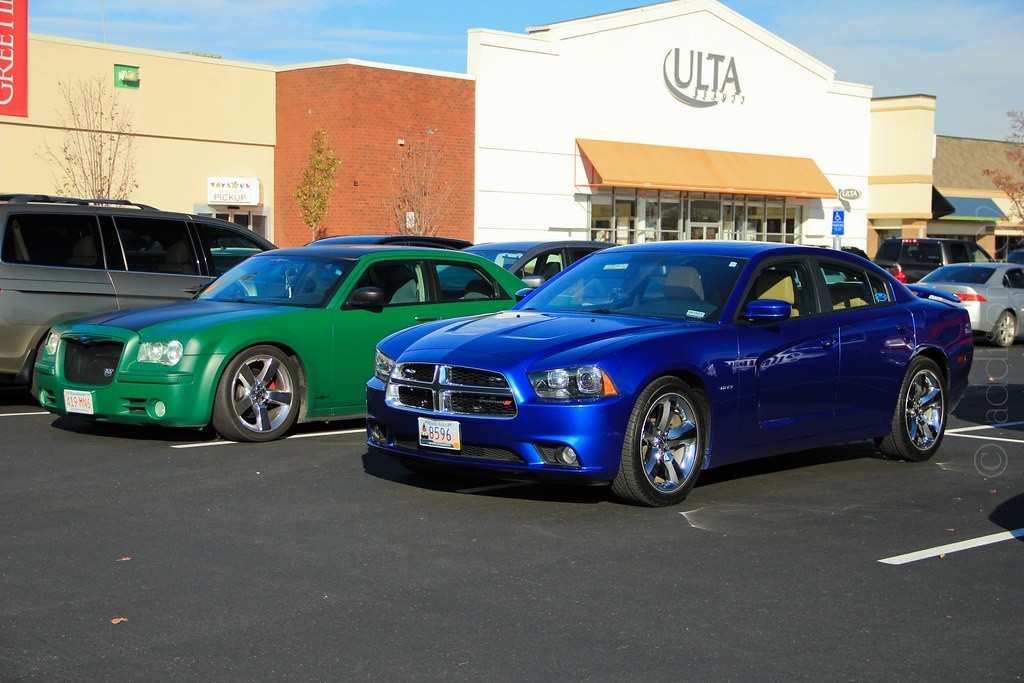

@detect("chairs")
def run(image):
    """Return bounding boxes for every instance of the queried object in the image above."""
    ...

[378,274,418,303]
[67,236,97,267]
[659,266,705,303]
[829,283,867,310]
[464,280,492,299]
[162,243,195,275]
[755,270,800,317]
[540,262,559,279]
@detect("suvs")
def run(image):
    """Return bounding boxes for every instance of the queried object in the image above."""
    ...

[873,237,995,283]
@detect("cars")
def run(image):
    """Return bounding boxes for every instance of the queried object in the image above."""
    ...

[305,235,474,250]
[462,240,616,290]
[365,240,975,508]
[32,246,535,443]
[917,263,1024,347]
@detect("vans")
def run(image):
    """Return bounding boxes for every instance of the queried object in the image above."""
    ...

[0,193,279,385]
